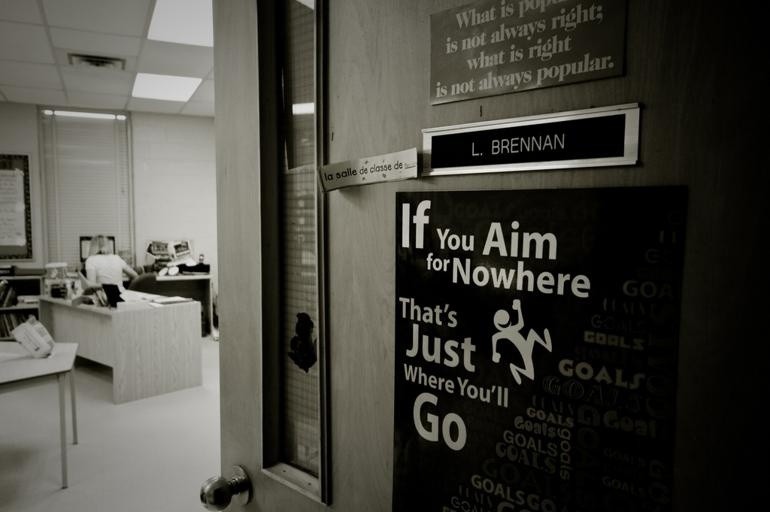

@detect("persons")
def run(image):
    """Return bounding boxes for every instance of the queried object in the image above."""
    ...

[85,233,141,292]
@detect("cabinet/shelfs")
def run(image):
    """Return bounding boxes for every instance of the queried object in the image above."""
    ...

[0,275,46,341]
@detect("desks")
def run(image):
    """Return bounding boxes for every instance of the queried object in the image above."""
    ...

[124,274,213,340]
[0,340,79,489]
[38,287,203,405]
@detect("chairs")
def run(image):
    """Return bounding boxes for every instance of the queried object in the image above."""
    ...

[128,272,157,292]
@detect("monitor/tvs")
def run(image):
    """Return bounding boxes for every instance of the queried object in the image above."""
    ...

[79,235,115,262]
